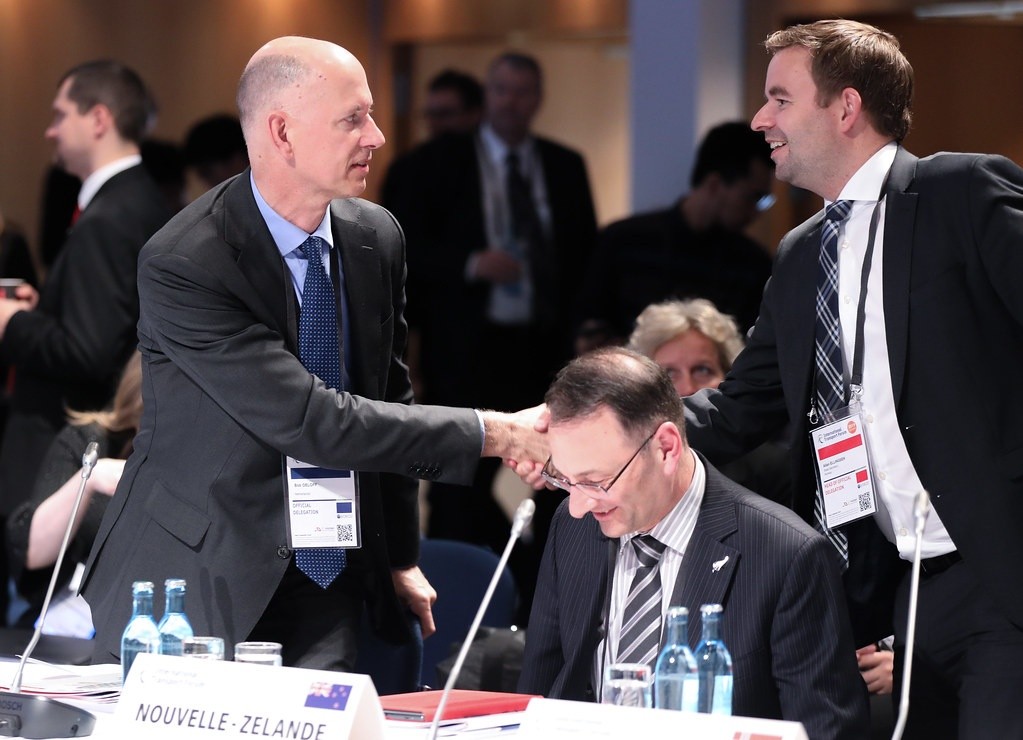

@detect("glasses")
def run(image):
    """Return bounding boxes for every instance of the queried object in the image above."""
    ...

[724,174,778,213]
[540,423,664,494]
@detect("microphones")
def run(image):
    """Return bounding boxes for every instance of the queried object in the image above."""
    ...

[428,499,537,740]
[0,442,101,740]
[891,491,931,740]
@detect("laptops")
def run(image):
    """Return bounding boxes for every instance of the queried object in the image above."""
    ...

[378,689,543,723]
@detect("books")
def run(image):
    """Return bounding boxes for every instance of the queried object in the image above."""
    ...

[378,689,544,737]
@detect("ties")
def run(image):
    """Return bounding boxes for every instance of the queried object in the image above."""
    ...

[812,199,850,580]
[296,237,346,590]
[506,152,551,315]
[615,535,668,707]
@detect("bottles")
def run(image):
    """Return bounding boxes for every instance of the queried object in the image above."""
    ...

[121,580,162,685]
[655,608,698,715]
[0,278,24,298]
[158,578,194,656]
[694,604,733,716]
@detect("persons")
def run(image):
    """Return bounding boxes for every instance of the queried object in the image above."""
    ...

[78,36,557,696]
[0,49,775,606]
[515,345,872,740]
[503,21,1023,740]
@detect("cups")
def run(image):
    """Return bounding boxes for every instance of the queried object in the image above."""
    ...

[608,663,652,709]
[182,636,225,660]
[234,641,282,666]
